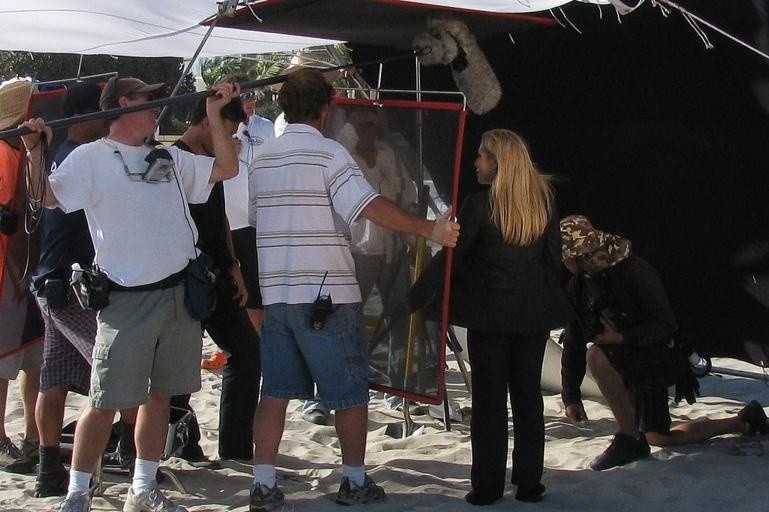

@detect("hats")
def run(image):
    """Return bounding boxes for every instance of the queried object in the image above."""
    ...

[61,82,102,118]
[99,77,168,111]
[560,216,631,270]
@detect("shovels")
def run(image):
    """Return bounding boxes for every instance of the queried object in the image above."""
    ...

[385,185,430,439]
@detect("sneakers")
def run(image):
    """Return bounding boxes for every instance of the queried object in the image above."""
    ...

[589,431,651,470]
[0,432,189,512]
[397,400,425,415]
[335,472,387,505]
[738,399,769,436]
[303,409,327,425]
[249,481,284,512]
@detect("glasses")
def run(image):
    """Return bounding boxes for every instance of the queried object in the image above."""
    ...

[240,90,255,101]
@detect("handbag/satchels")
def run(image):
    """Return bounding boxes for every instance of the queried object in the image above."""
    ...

[184,262,218,321]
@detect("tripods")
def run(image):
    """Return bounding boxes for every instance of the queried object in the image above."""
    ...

[365,252,474,434]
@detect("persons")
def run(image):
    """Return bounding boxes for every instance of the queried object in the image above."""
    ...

[557,214,769,471]
[0,67,460,512]
[452,129,565,506]
